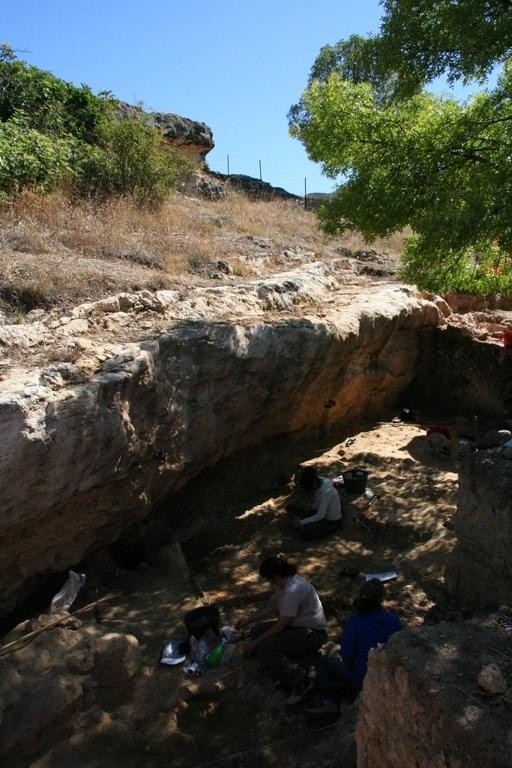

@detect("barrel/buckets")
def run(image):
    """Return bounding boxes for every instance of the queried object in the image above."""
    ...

[184,607,218,640]
[343,470,368,491]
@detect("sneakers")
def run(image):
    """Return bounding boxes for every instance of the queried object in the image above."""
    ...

[287,679,314,705]
[305,698,330,712]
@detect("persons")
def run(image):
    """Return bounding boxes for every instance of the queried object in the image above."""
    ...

[286,466,343,540]
[236,555,326,705]
[303,577,403,716]
